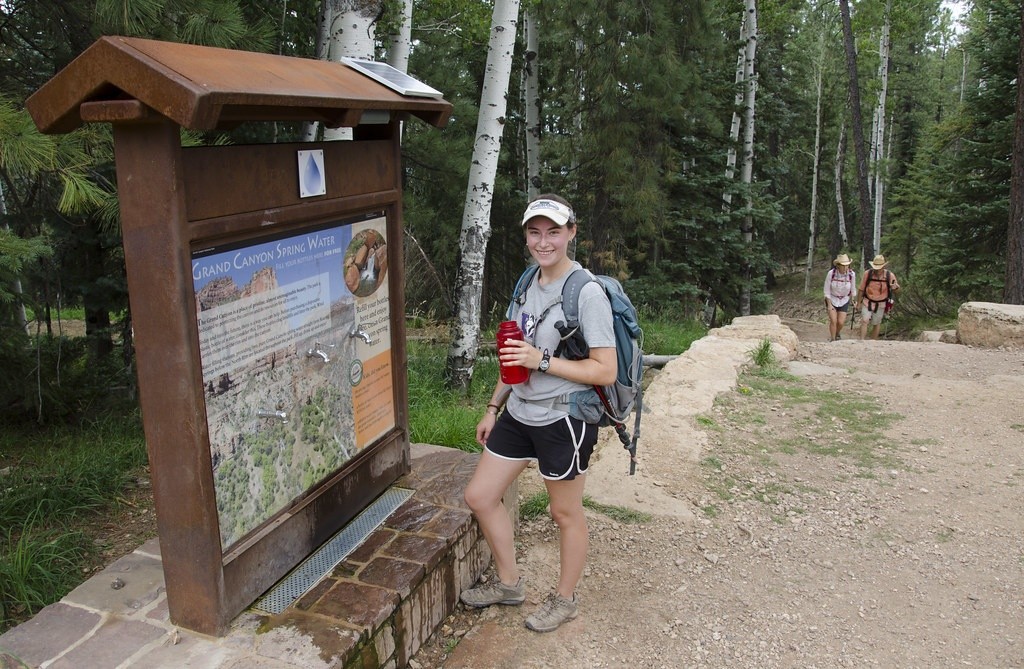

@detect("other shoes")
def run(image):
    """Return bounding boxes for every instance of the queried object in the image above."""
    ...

[836,335,841,340]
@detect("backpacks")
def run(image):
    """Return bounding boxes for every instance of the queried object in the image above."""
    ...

[509,263,643,427]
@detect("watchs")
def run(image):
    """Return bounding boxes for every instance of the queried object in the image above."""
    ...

[538,354,551,373]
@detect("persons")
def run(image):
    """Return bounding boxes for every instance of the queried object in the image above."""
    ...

[458,192,619,632]
[856,255,900,340]
[824,254,856,343]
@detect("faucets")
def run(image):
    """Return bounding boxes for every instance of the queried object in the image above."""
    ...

[348,321,375,345]
[306,341,336,364]
[255,410,289,426]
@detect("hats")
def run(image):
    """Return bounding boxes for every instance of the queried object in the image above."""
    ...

[869,255,889,269]
[522,200,577,225]
[832,254,853,266]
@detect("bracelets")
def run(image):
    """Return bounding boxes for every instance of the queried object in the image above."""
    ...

[485,408,497,416]
[487,403,501,413]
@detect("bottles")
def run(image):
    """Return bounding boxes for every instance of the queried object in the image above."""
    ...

[494,320,529,385]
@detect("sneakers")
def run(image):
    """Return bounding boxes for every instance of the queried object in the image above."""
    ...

[460,575,524,607]
[524,590,579,632]
